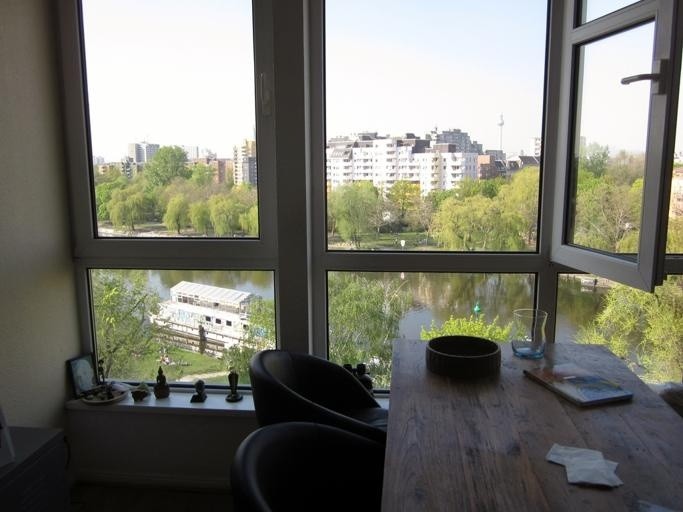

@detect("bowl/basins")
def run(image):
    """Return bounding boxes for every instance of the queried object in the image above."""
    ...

[425,335,499,379]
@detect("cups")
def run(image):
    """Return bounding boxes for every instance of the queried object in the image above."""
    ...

[511,309,548,358]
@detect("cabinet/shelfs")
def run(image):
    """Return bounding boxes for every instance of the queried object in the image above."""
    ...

[0,424,63,512]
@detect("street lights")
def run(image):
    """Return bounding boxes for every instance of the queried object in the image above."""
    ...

[473,301,483,323]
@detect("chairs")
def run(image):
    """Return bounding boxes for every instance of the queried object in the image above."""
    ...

[248,349,388,445]
[230,420,384,511]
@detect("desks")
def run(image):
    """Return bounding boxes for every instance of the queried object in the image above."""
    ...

[379,331,682,510]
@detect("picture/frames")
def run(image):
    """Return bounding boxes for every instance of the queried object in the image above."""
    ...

[65,351,99,399]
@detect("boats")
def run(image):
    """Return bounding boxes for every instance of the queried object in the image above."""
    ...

[146,279,275,358]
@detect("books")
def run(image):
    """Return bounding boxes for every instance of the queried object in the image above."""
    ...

[523,361,632,407]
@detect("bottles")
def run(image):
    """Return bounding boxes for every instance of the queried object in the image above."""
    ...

[344,363,373,396]
